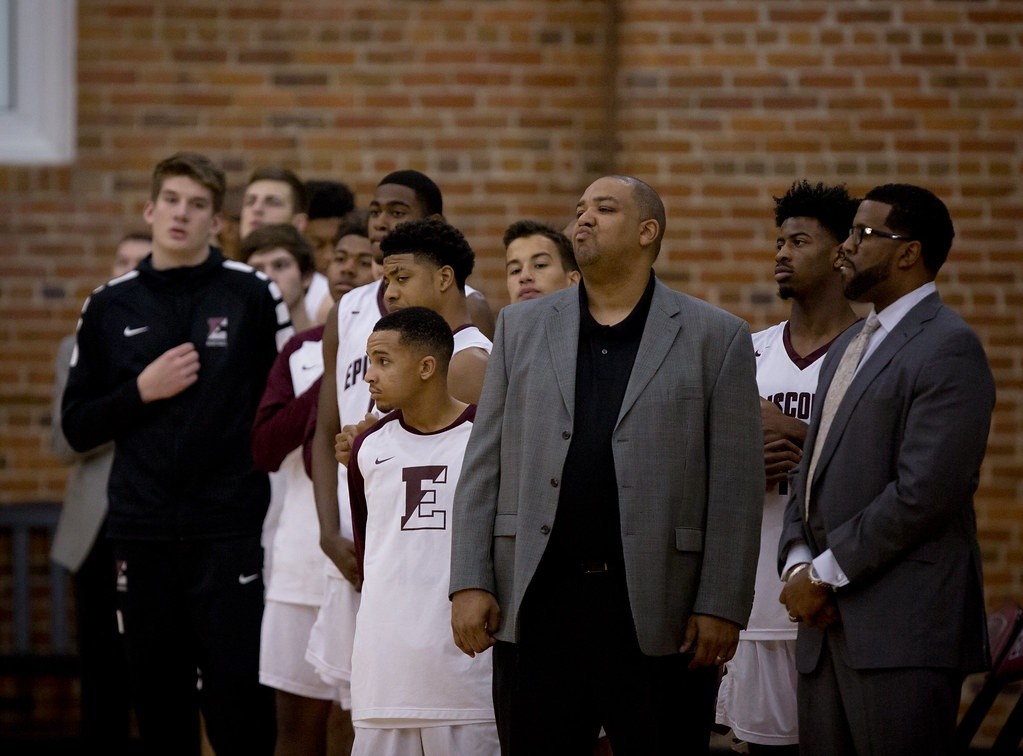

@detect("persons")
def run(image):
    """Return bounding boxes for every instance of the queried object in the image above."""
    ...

[347,305,502,756]
[777,183,997,756]
[504,220,580,305]
[49,166,494,756]
[449,174,765,755]
[716,181,864,756]
[59,152,296,756]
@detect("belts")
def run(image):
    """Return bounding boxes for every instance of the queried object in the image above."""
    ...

[538,554,627,580]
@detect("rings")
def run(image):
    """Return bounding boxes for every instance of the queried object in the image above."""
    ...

[788,611,797,621]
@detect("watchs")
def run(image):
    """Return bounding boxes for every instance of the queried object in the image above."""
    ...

[808,564,831,588]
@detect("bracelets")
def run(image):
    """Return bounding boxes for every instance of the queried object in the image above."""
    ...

[788,564,807,580]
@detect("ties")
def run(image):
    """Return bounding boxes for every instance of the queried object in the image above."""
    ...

[804,311,881,522]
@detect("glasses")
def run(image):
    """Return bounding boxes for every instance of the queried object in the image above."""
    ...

[849,225,914,246]
